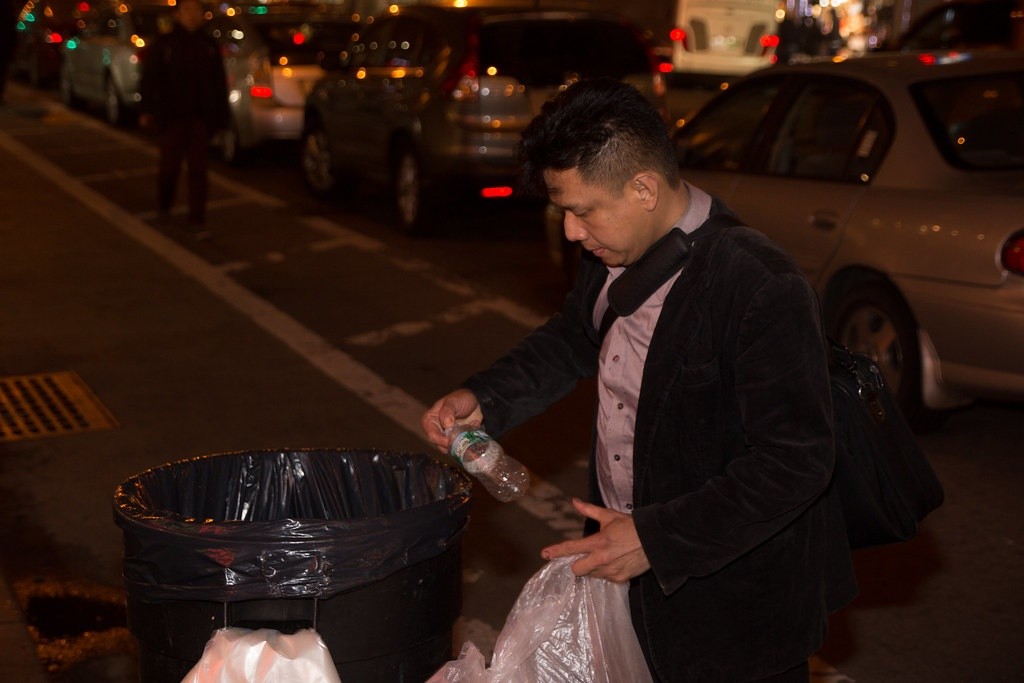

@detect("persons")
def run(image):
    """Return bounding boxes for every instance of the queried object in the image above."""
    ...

[139,0,231,235]
[422,81,837,683]
[772,8,840,58]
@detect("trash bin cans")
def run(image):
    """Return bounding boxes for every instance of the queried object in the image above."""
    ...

[113,446,473,683]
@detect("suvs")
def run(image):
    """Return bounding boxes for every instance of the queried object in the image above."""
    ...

[304,7,670,234]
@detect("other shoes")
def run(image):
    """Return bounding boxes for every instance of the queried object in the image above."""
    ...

[158,200,181,219]
[192,229,212,243]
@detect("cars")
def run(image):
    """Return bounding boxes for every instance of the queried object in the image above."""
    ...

[60,16,156,125]
[192,14,327,165]
[668,50,1023,396]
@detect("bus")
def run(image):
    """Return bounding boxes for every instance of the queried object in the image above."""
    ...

[671,0,786,80]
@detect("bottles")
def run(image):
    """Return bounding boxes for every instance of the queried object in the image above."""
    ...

[443,420,531,500]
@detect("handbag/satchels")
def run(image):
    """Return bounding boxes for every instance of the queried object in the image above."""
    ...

[827,332,946,550]
